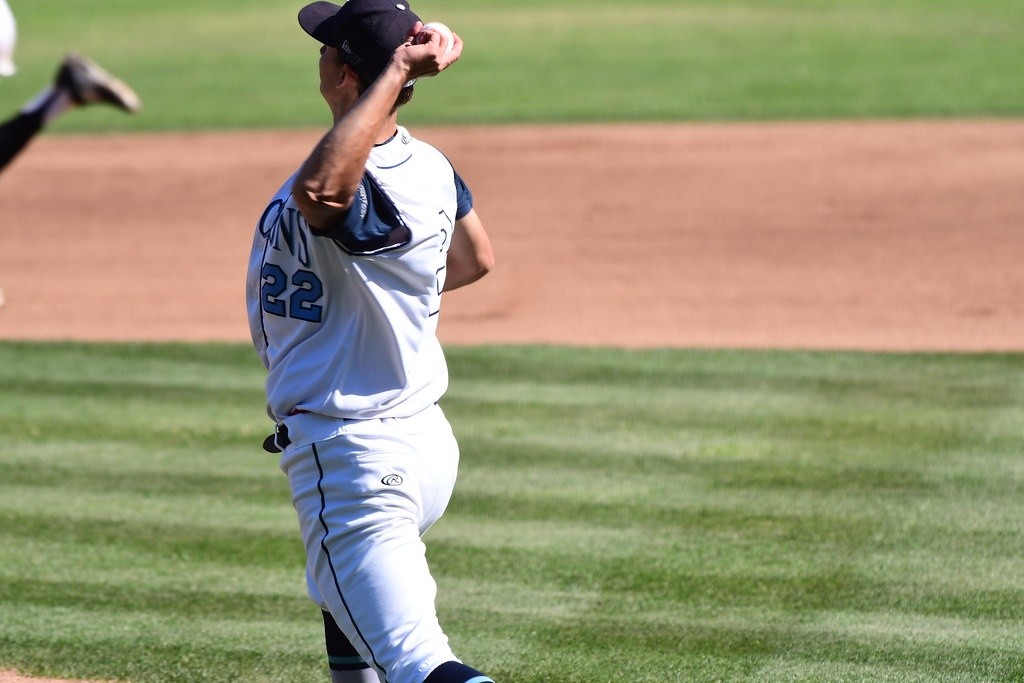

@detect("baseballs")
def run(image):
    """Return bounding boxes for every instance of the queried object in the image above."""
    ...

[415,21,455,53]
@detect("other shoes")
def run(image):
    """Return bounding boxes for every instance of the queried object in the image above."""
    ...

[56,55,140,113]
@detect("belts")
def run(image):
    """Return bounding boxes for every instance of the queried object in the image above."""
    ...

[263,417,354,453]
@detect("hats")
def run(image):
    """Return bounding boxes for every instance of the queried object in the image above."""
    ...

[298,0,424,86]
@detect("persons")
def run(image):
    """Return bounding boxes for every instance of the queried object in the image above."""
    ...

[0,0,141,174]
[246,0,494,683]
